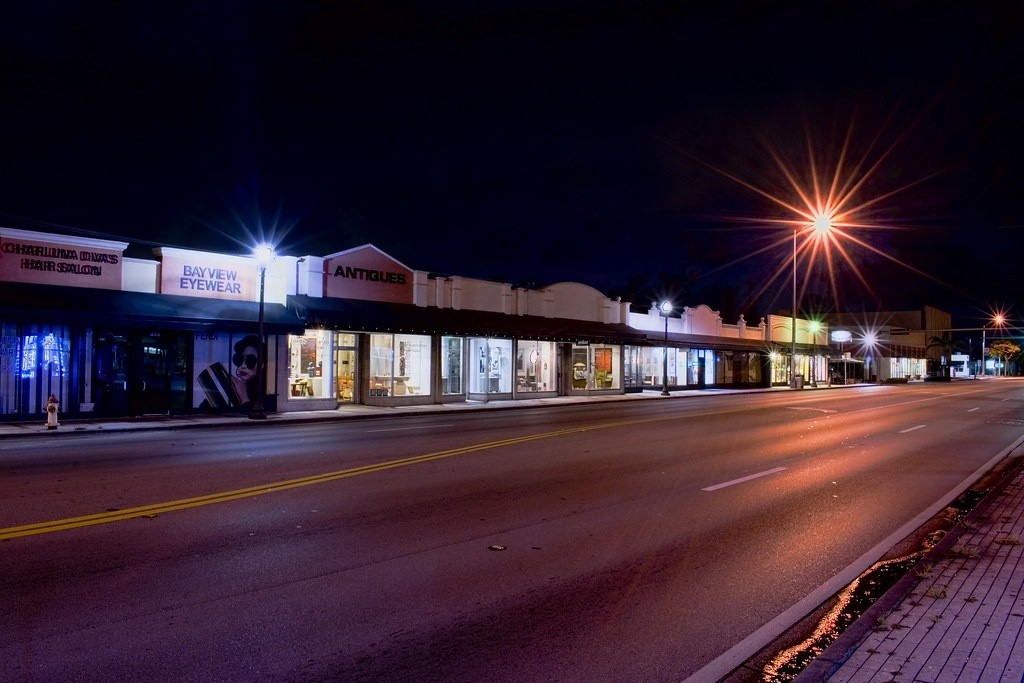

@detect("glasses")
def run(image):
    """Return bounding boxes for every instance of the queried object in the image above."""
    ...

[233,353,258,369]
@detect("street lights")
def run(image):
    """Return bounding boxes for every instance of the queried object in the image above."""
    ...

[789,217,833,389]
[249,247,272,420]
[982,318,1003,377]
[868,337,873,383]
[661,301,671,396]
[810,324,818,387]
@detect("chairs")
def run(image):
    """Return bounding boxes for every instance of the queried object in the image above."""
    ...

[596,369,614,389]
[668,376,677,385]
[573,369,587,390]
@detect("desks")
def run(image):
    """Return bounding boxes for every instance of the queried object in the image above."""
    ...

[291,384,306,397]
[372,376,410,395]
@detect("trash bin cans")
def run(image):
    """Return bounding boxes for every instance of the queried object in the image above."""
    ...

[795,375,804,388]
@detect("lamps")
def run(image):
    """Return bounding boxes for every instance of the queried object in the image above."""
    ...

[574,363,586,370]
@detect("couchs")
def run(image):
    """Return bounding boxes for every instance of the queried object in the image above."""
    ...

[642,376,659,385]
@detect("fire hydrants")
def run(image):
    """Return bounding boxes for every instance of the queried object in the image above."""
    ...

[44,393,60,430]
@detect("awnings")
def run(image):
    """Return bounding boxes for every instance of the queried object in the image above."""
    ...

[771,340,844,355]
[287,294,647,346]
[638,330,788,354]
[0,280,306,337]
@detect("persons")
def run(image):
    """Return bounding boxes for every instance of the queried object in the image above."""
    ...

[199,335,267,408]
